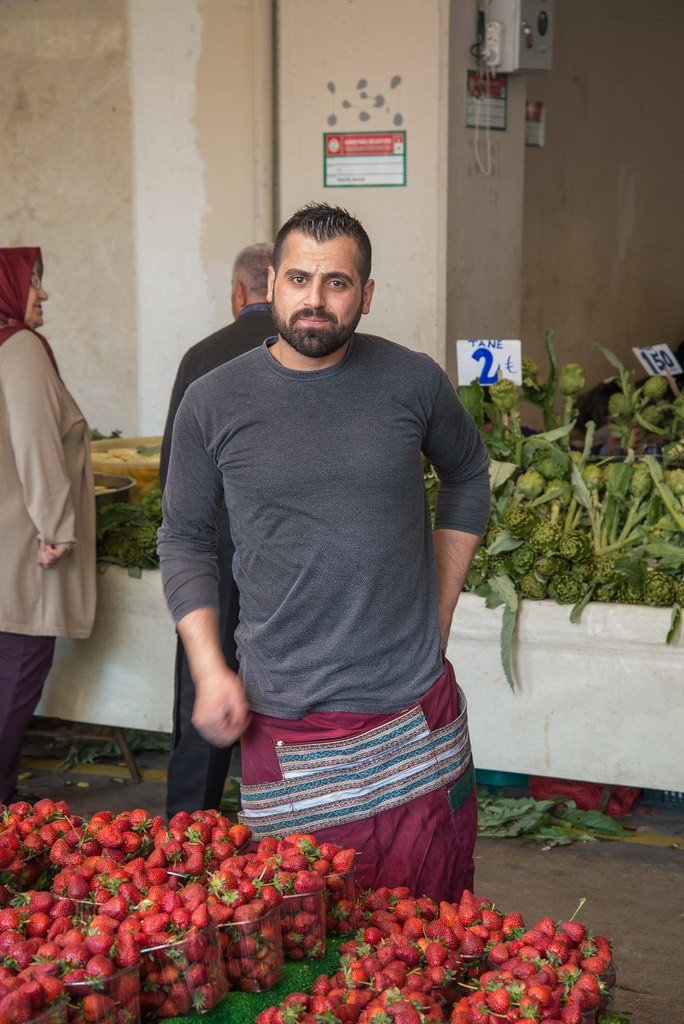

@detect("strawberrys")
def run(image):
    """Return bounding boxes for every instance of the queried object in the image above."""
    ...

[0,797,615,1024]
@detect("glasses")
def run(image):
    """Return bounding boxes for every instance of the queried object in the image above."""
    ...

[31,275,41,288]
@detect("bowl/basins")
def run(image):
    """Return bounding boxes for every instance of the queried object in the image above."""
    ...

[93,472,138,509]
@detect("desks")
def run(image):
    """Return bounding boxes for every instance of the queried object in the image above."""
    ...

[31,566,684,813]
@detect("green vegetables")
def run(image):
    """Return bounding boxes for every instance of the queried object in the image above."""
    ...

[58,729,170,773]
[475,788,633,846]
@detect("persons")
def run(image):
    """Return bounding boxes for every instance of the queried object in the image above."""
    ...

[166,244,279,818]
[0,245,99,797]
[161,207,491,903]
